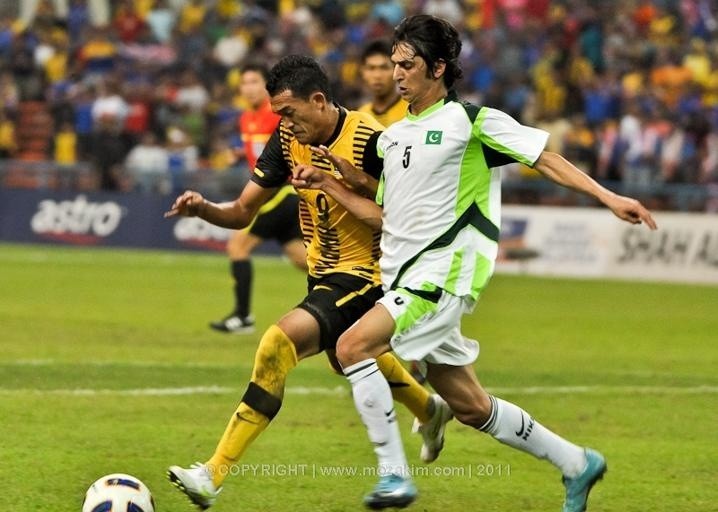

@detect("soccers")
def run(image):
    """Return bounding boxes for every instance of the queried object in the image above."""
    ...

[83,472,154,511]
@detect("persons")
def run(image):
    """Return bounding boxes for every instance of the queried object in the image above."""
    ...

[209,61,310,330]
[163,56,459,512]
[291,16,659,510]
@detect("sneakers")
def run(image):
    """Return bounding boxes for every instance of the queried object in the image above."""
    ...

[364,474,416,511]
[210,313,256,332]
[166,465,222,510]
[410,359,429,385]
[563,446,608,512]
[412,392,452,463]
